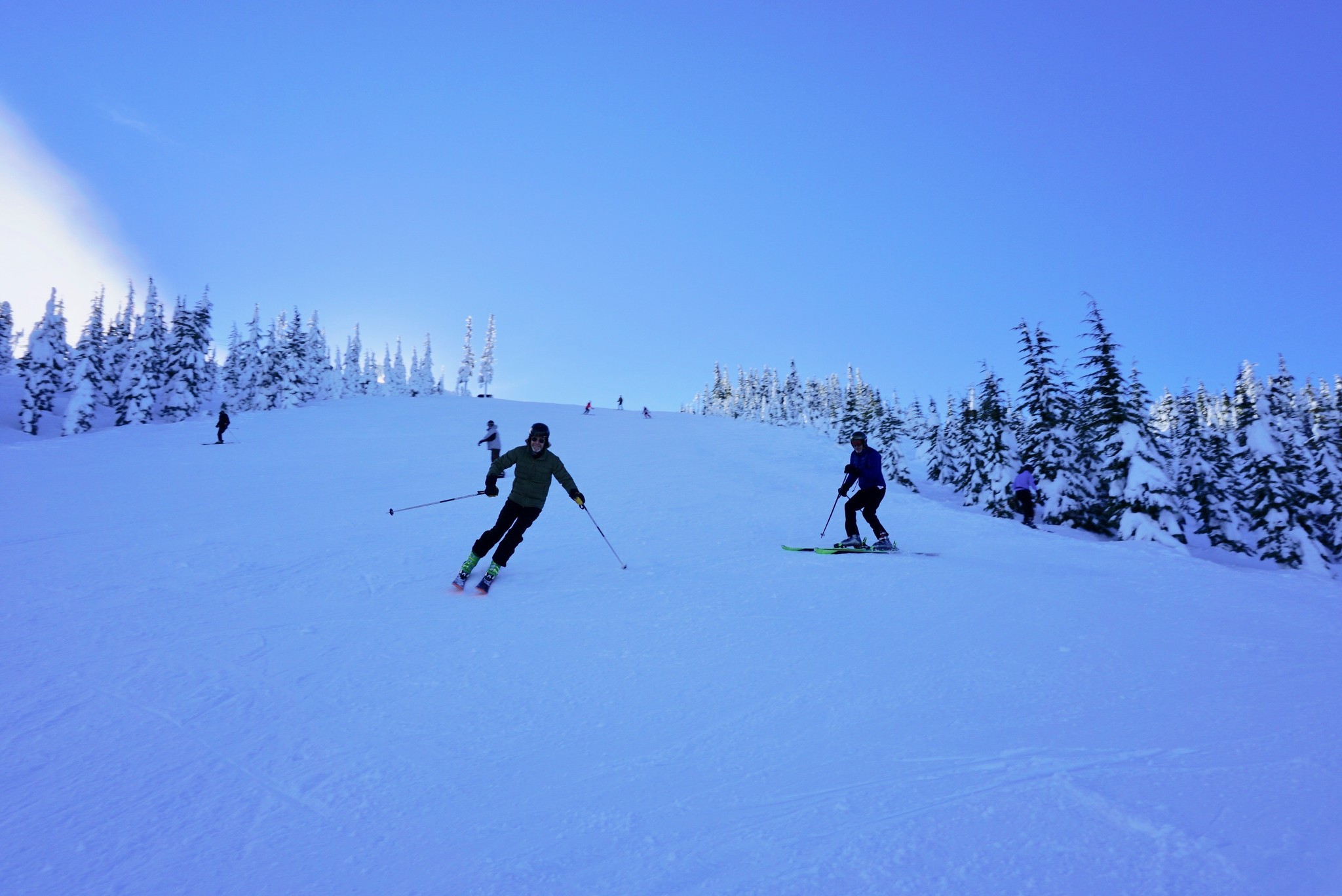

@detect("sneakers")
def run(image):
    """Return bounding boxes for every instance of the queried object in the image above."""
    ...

[834,536,862,548]
[486,560,500,576]
[461,551,481,574]
[873,539,892,549]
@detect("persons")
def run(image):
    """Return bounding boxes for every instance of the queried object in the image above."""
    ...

[478,420,505,478]
[838,432,892,547]
[641,408,651,418]
[461,423,585,576]
[584,400,594,414]
[617,395,623,410]
[216,411,230,443]
[1012,464,1041,529]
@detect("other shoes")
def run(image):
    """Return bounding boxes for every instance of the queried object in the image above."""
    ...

[1028,522,1035,528]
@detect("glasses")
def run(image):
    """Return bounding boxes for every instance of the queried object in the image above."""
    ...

[851,439,862,447]
[530,437,546,443]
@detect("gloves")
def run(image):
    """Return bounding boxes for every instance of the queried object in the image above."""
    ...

[570,489,585,505]
[838,485,849,497]
[485,485,499,498]
[844,464,855,474]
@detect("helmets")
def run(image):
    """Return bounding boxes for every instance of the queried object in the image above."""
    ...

[850,432,867,446]
[488,421,494,426]
[529,423,549,438]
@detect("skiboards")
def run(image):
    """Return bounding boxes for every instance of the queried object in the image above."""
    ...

[780,544,939,557]
[451,560,495,593]
